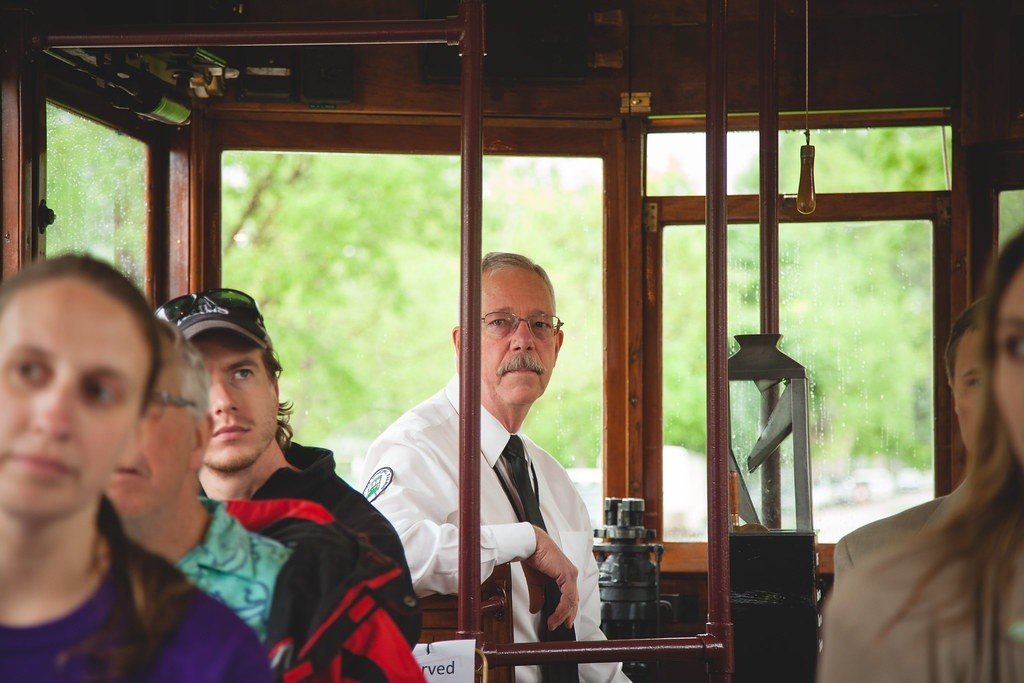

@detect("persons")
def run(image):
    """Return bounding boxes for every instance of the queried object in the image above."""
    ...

[817,227,1022,683]
[103,289,428,683]
[358,253,634,683]
[1,250,274,682]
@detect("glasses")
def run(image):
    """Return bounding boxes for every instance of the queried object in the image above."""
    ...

[478,311,564,340]
[141,393,202,423]
[152,288,267,330]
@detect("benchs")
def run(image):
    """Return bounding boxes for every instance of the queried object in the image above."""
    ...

[417,561,518,682]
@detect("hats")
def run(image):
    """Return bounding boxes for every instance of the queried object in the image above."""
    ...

[160,291,274,350]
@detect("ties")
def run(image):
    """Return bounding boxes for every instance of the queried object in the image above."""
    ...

[502,434,580,683]
